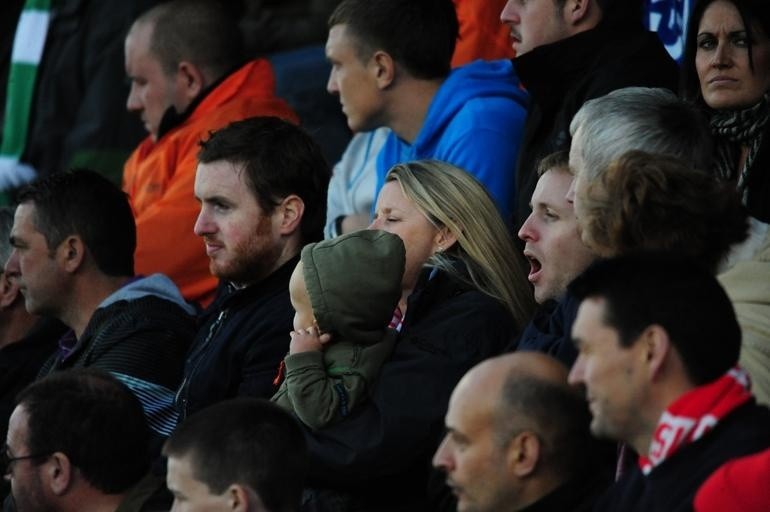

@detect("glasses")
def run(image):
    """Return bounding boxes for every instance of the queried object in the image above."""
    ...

[0,449,53,475]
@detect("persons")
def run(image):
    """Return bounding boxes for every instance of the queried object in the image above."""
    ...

[0,1,769,511]
